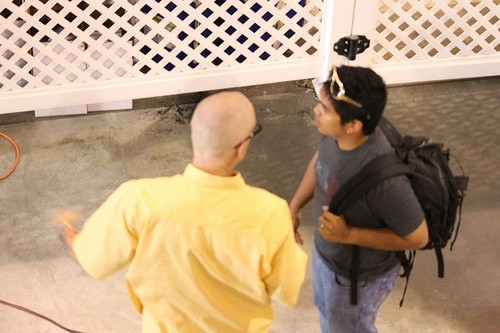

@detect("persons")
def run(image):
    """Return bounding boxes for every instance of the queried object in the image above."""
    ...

[273,63,428,333]
[57,90,307,332]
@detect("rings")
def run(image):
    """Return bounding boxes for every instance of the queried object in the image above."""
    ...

[320,224,324,229]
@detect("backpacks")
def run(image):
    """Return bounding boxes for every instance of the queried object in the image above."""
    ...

[327,135,469,310]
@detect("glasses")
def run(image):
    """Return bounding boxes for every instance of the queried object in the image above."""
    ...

[328,64,372,121]
[234,123,264,150]
